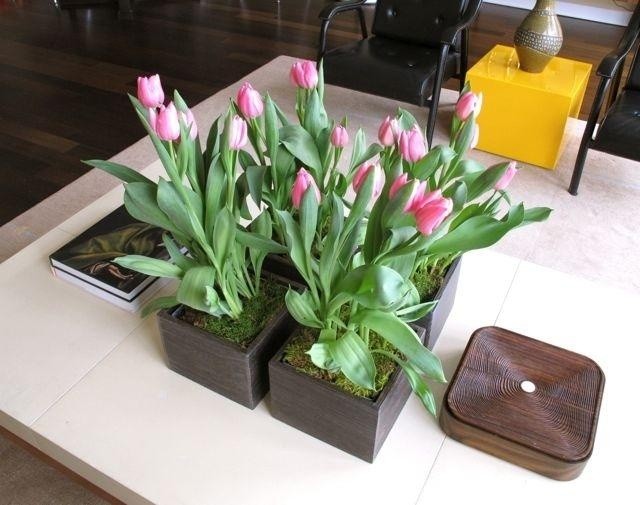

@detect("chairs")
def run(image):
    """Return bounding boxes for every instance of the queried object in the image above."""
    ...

[569,1,640,195]
[308,1,483,154]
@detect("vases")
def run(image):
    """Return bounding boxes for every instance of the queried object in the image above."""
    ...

[346,253,458,352]
[268,309,428,465]
[516,1,565,75]
[244,207,349,286]
[155,273,296,410]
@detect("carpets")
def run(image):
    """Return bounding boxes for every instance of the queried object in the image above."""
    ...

[1,55,640,504]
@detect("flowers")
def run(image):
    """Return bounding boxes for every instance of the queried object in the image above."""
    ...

[85,72,320,310]
[337,91,555,298]
[240,58,367,250]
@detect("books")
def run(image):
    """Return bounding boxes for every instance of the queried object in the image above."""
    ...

[49,254,192,314]
[49,202,189,302]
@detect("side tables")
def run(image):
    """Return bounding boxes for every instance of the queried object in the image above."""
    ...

[463,45,592,173]
